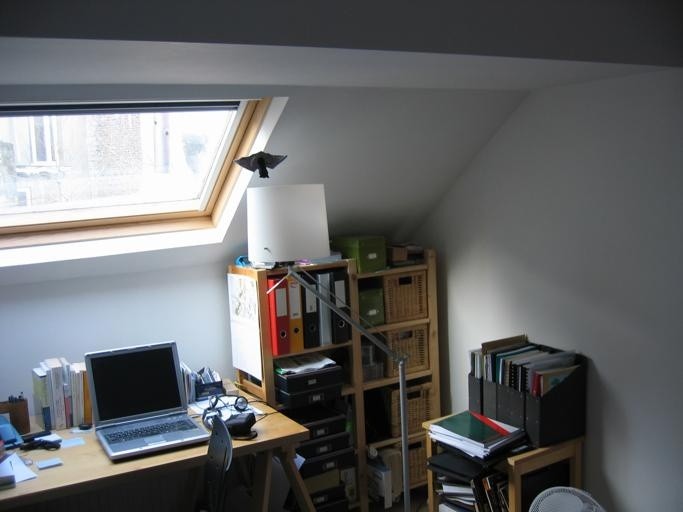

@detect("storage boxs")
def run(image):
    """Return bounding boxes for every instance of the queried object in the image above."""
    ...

[332,234,387,275]
[359,288,385,329]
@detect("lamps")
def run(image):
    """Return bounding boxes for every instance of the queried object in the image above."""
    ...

[246,183,411,512]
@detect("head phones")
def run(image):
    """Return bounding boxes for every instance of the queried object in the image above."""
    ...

[207,393,250,412]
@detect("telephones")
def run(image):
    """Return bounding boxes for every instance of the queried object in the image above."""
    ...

[0,416,22,451]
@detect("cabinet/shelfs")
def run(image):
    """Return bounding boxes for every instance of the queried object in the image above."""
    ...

[422,409,587,512]
[228,248,444,512]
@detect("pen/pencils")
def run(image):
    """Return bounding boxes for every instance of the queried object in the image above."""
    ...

[7,392,25,402]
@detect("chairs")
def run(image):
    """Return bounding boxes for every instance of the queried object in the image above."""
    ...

[192,415,232,512]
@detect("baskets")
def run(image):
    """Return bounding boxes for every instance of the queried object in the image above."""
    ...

[394,435,427,486]
[385,324,430,377]
[384,270,427,322]
[379,382,432,436]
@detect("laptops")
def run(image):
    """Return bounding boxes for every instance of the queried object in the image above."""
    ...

[84,340,210,463]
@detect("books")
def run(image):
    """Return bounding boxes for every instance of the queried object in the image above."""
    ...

[0,482,16,490]
[180,360,195,404]
[0,460,16,485]
[428,410,529,469]
[428,452,510,511]
[33,357,94,430]
[467,333,582,399]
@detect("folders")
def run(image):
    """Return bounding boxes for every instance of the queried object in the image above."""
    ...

[269,271,350,357]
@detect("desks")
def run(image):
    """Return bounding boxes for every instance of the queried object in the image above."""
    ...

[0,387,316,512]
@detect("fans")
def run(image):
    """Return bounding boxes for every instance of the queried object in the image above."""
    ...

[529,486,607,512]
